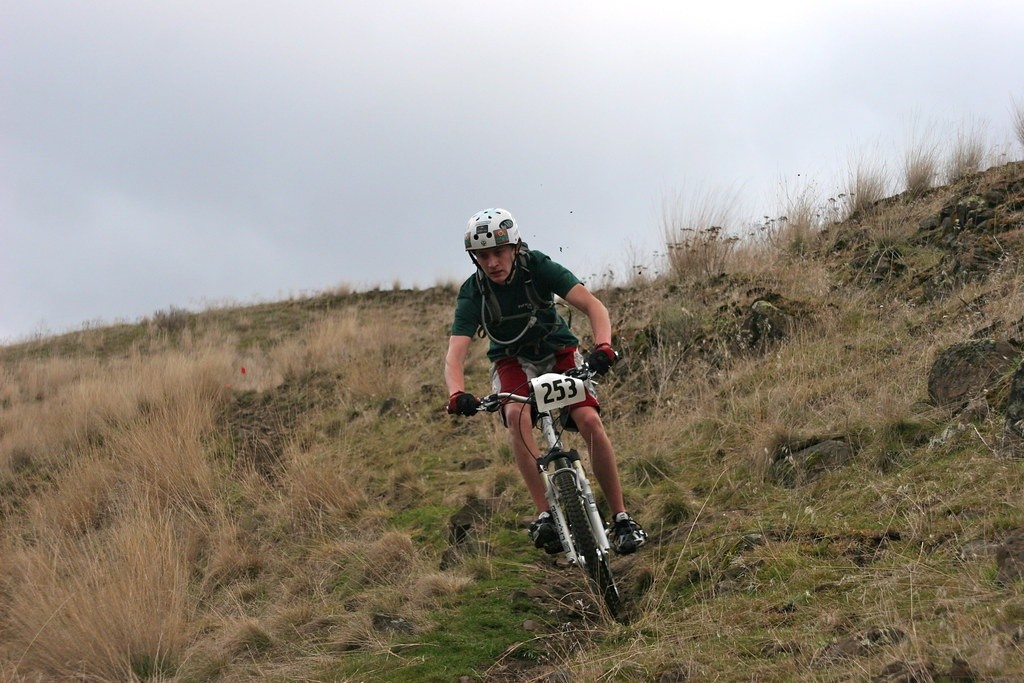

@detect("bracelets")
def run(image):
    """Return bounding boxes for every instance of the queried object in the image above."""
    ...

[595,342,610,349]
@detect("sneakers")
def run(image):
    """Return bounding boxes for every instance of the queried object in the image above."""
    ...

[527,512,566,555]
[613,512,648,556]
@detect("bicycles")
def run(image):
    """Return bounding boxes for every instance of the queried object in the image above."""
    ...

[444,350,633,629]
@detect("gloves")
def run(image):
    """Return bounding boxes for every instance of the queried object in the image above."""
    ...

[588,343,617,376]
[449,390,479,417]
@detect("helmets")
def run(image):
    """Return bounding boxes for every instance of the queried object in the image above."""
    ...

[462,207,523,251]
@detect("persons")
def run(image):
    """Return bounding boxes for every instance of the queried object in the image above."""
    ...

[445,208,648,555]
